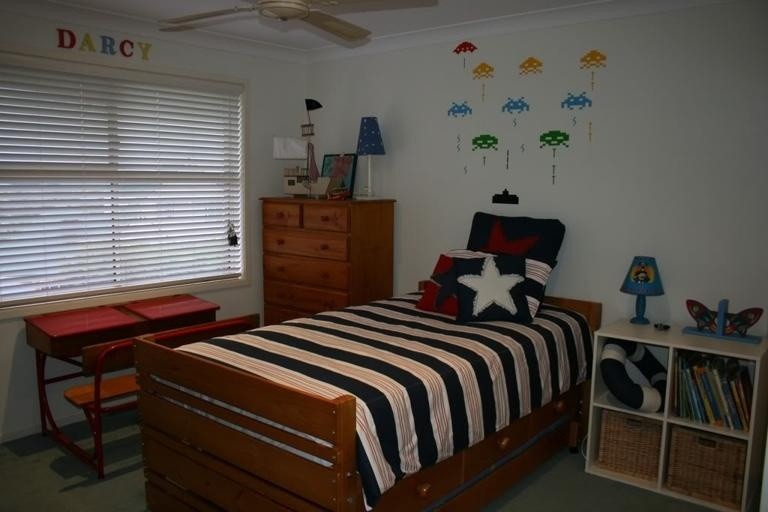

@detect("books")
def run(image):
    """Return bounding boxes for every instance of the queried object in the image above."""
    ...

[672,349,752,432]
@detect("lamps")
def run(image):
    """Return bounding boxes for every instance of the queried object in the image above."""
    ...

[620,255,664,324]
[356,116,387,197]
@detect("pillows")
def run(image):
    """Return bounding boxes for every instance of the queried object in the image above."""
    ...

[417,253,481,317]
[467,210,565,314]
[456,252,534,323]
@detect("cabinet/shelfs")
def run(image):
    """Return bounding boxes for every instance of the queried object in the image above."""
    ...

[584,317,768,512]
[258,195,396,324]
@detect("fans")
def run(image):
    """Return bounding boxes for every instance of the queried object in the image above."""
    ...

[156,1,438,48]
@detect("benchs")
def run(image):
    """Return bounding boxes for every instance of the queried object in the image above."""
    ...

[64,312,261,479]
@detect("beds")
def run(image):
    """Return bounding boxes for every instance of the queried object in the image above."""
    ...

[135,277,604,512]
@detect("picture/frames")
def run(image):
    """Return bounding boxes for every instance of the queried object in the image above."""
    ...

[320,153,357,198]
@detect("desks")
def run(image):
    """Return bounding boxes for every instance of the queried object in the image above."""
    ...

[24,293,222,466]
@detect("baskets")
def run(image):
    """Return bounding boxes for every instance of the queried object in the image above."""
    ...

[665,424,746,510]
[596,409,662,483]
